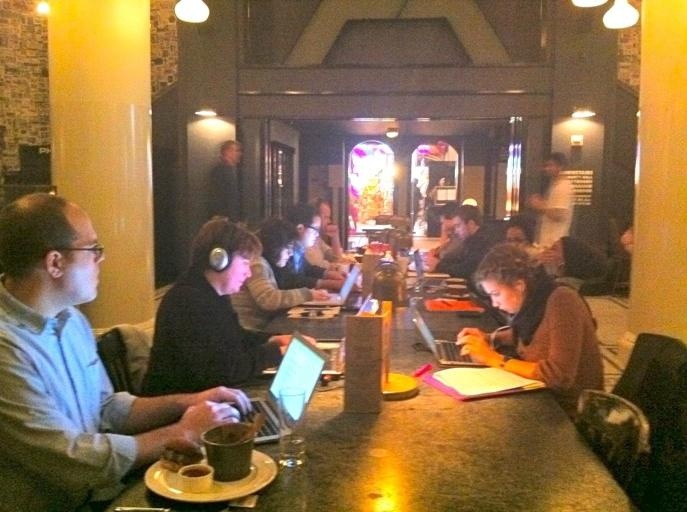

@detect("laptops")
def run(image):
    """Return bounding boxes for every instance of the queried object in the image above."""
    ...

[412,249,444,288]
[259,291,376,380]
[304,265,360,305]
[227,331,327,446]
[410,305,485,366]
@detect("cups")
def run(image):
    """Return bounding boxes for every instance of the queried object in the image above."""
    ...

[276,386,309,469]
[200,422,255,483]
[176,461,214,493]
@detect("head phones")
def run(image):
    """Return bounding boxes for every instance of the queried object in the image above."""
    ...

[210,223,234,272]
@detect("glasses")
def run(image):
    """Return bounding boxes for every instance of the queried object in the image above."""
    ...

[43,243,105,263]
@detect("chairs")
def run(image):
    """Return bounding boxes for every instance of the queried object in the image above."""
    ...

[610,330,686,511]
[95,327,133,394]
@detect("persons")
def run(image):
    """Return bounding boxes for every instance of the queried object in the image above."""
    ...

[529,152,576,248]
[137,198,361,399]
[455,244,605,421]
[2,193,252,510]
[421,201,601,308]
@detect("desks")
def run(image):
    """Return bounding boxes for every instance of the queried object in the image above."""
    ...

[110,249,640,510]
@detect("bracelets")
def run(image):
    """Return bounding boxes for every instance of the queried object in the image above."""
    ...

[500,355,513,369]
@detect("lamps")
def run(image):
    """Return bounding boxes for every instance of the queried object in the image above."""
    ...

[385,127,399,138]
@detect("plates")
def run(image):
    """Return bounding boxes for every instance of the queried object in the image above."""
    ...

[141,444,279,504]
